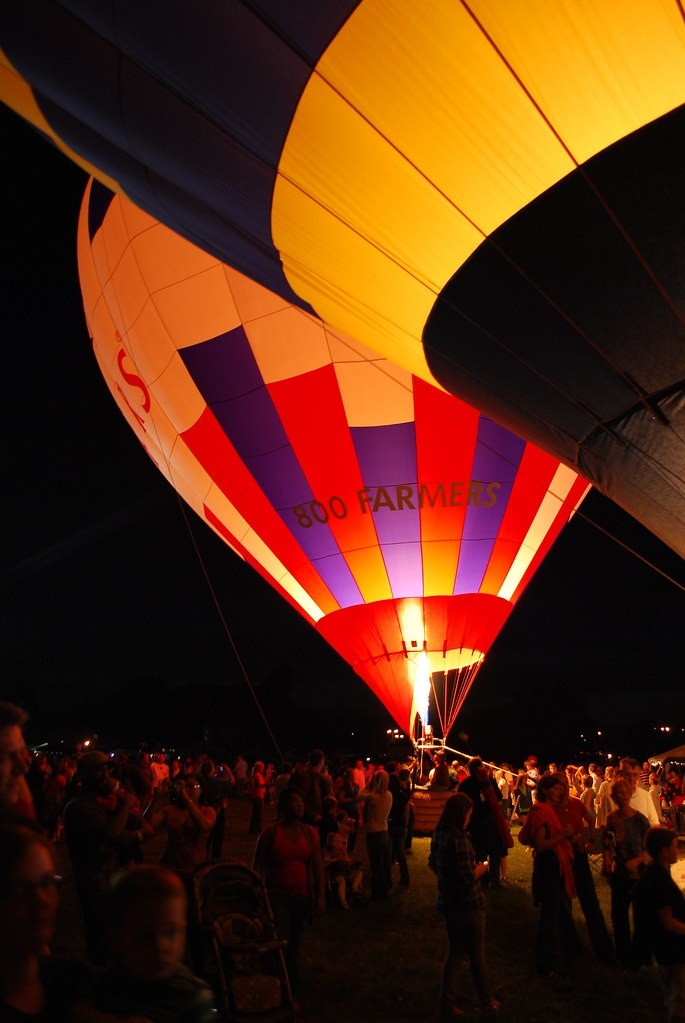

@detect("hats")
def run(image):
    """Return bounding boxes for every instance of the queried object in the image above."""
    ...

[77,751,115,774]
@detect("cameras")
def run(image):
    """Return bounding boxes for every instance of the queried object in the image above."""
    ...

[167,783,180,792]
[216,766,224,772]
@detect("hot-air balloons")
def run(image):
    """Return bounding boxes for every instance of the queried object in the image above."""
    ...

[0,1,684,595]
[74,162,594,838]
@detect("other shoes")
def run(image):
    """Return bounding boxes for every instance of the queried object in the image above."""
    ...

[339,900,351,910]
[600,958,615,968]
[440,1007,467,1022]
[482,997,502,1013]
[537,969,563,982]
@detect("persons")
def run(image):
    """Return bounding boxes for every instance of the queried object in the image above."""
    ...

[94,865,220,1023]
[0,703,685,1023]
[633,827,685,1022]
[253,789,325,1014]
[428,794,502,1019]
